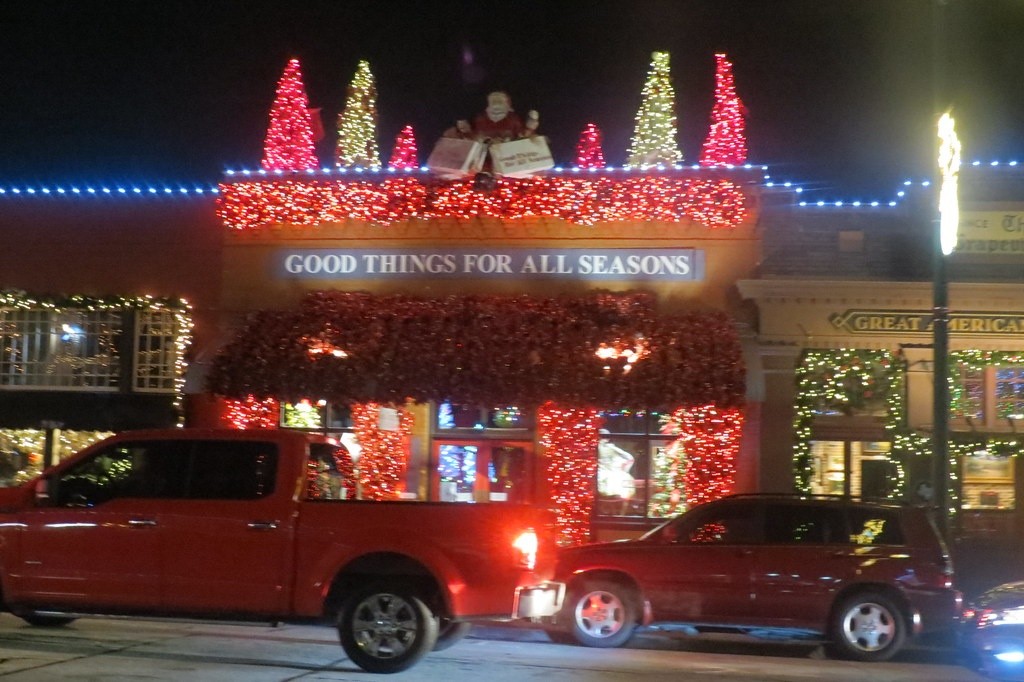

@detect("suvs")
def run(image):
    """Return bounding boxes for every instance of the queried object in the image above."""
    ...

[543,488,966,665]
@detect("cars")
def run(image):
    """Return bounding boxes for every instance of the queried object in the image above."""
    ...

[958,581,1024,680]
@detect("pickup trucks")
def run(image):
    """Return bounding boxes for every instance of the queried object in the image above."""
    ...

[2,423,563,677]
